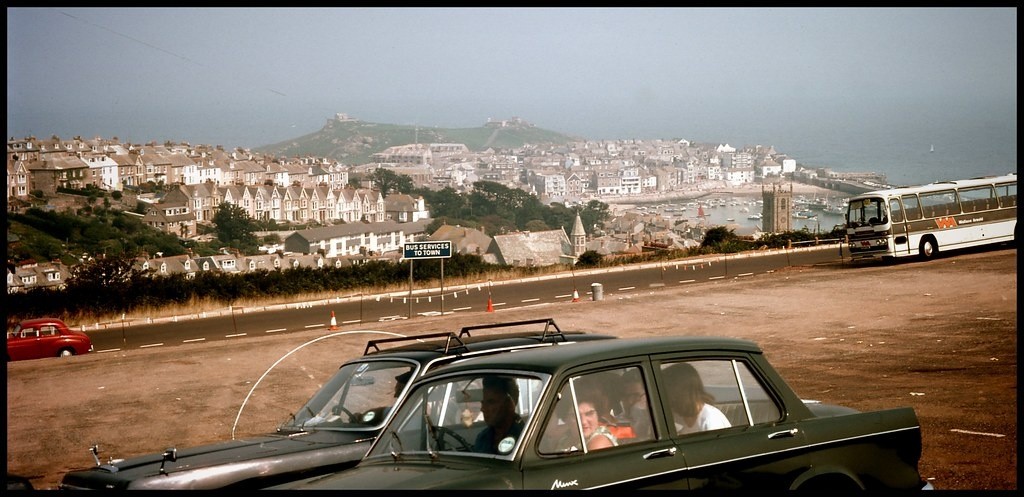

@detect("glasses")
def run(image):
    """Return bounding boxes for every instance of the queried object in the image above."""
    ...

[624,392,645,403]
[479,395,506,406]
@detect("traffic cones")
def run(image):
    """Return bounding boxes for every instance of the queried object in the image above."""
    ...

[485,298,495,313]
[328,312,341,331]
[572,289,581,303]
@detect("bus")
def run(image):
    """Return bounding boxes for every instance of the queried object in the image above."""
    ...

[844,173,1016,262]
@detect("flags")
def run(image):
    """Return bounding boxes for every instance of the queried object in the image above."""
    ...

[698,206,706,221]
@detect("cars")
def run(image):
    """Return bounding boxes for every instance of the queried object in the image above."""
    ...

[4,318,92,362]
[59,318,632,497]
[258,336,924,494]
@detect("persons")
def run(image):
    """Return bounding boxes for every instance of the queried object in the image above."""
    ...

[555,380,619,455]
[348,371,414,425]
[6,264,16,286]
[582,363,732,439]
[474,376,527,456]
